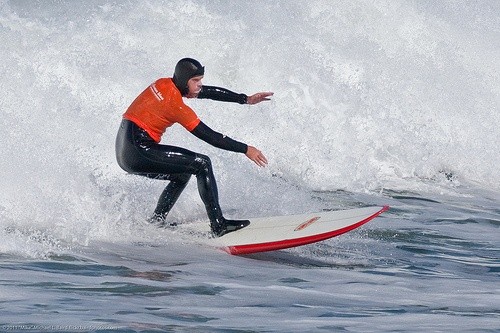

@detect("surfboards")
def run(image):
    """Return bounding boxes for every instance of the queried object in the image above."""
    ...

[174,205,389,254]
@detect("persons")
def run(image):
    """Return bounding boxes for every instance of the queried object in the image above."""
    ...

[115,57,274,239]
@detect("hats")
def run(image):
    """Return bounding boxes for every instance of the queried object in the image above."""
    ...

[175,57,204,89]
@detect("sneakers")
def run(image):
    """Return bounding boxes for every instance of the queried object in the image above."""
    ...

[211,215,250,238]
[169,222,177,225]
